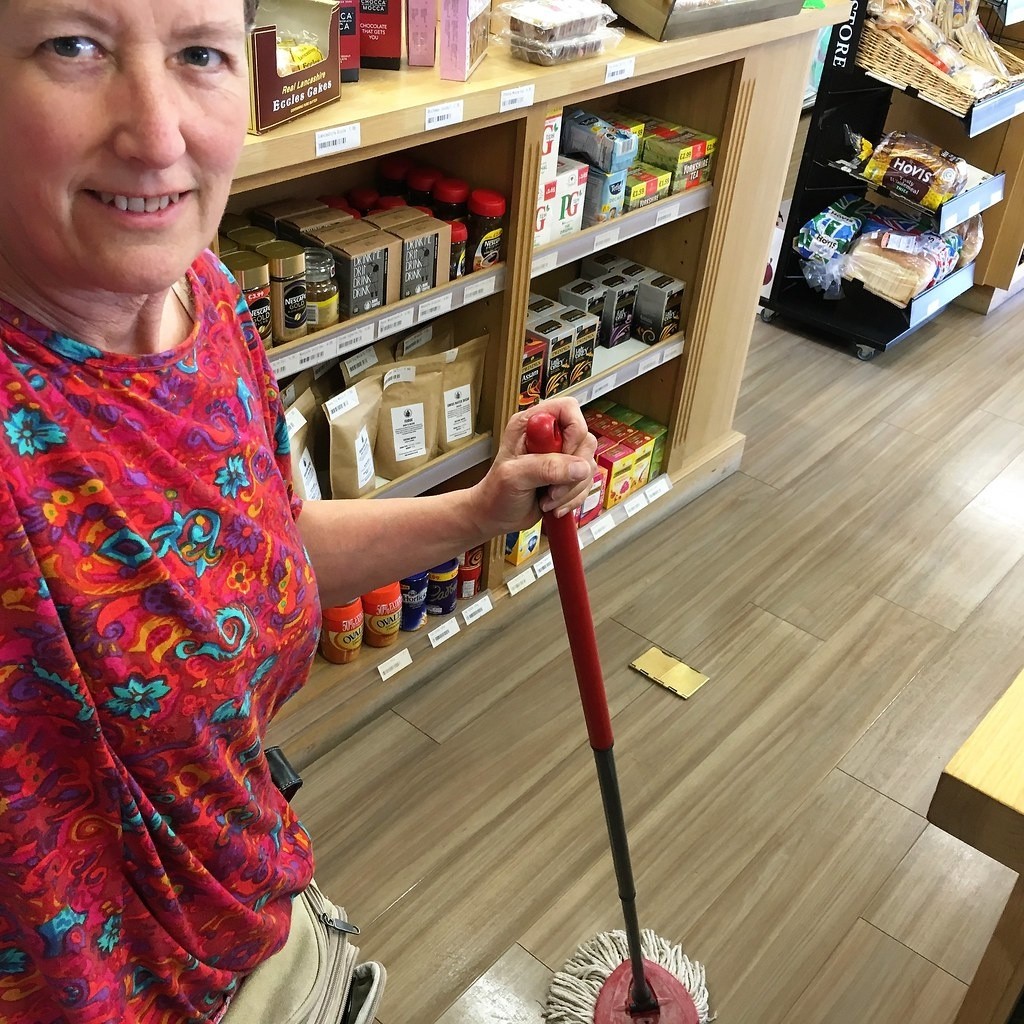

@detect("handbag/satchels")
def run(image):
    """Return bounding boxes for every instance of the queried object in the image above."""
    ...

[221,877,386,1023]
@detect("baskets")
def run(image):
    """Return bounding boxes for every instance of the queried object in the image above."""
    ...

[857,21,1023,110]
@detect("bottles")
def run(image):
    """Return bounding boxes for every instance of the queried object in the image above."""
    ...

[328,164,506,289]
[217,205,339,356]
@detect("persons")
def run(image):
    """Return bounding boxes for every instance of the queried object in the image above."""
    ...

[0,1,600,1024]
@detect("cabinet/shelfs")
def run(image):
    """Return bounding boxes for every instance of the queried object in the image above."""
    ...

[753,0,1023,364]
[210,0,854,769]
